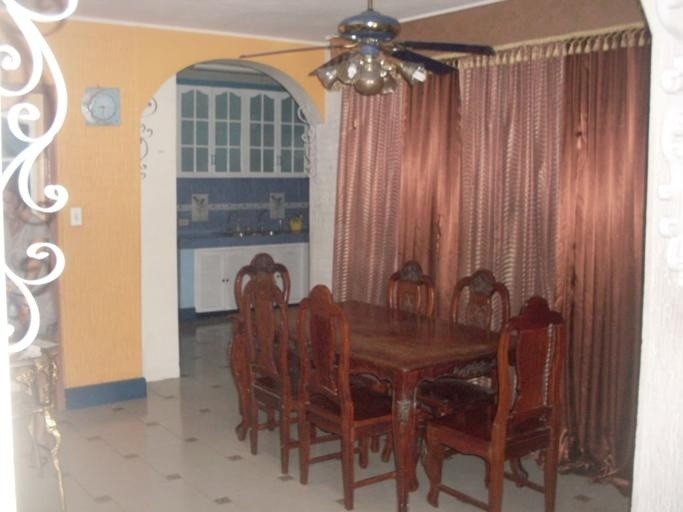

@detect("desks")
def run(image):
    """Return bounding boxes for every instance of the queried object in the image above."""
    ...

[221,297,562,508]
[6,323,69,512]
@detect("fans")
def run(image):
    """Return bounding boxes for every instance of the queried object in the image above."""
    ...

[236,0,499,82]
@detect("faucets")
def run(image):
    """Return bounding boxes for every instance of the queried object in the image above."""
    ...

[256,209,269,225]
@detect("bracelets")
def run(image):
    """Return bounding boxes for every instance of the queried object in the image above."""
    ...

[23,302,29,308]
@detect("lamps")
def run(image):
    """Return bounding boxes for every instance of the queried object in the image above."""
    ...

[313,41,434,101]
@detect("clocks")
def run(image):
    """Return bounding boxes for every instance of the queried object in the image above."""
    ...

[77,85,121,129]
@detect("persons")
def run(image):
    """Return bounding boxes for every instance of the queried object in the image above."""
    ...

[3,170,69,470]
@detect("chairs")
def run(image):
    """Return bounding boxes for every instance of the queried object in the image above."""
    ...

[419,294,567,511]
[367,259,439,460]
[292,280,422,509]
[443,267,511,331]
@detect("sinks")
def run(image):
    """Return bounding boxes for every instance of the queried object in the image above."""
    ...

[224,230,282,239]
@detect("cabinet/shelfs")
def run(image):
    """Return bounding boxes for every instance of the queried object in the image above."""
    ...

[193,246,307,317]
[179,81,245,182]
[244,84,308,182]
